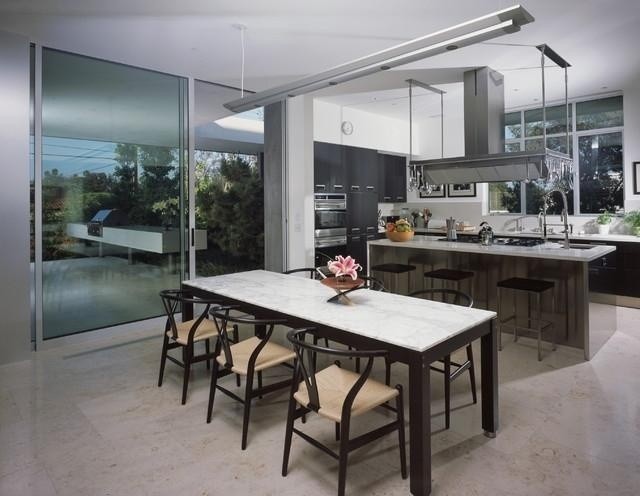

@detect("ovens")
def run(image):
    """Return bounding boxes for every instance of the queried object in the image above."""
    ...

[312,192,347,273]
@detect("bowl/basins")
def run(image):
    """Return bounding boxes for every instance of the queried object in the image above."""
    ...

[384,231,416,241]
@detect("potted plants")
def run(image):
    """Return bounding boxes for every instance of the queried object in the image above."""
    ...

[597,210,640,237]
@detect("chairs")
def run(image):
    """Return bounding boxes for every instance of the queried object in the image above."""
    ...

[206,305,305,450]
[158,287,239,405]
[385,288,478,429]
[279,268,331,372]
[321,273,390,374]
[281,325,409,495]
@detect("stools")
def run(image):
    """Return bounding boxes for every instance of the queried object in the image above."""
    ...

[371,264,556,364]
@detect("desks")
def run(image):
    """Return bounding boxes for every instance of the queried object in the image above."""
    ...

[181,267,501,496]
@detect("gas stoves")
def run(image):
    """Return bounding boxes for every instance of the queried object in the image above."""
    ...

[440,232,538,246]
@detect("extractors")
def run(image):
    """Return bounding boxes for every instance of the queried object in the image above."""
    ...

[411,67,577,187]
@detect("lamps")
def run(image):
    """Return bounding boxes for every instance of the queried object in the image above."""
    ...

[223,0,536,114]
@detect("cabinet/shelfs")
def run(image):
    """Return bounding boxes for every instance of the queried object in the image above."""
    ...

[348,192,379,271]
[590,243,639,297]
[314,141,379,194]
[379,154,407,203]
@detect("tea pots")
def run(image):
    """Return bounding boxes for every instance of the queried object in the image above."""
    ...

[478,222,494,245]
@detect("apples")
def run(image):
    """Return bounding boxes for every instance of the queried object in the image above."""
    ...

[386,224,395,233]
[396,225,405,232]
[405,224,410,230]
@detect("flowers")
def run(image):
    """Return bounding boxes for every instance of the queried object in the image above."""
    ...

[326,253,363,281]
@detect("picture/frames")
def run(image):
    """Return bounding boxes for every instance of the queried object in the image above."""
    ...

[418,175,476,199]
